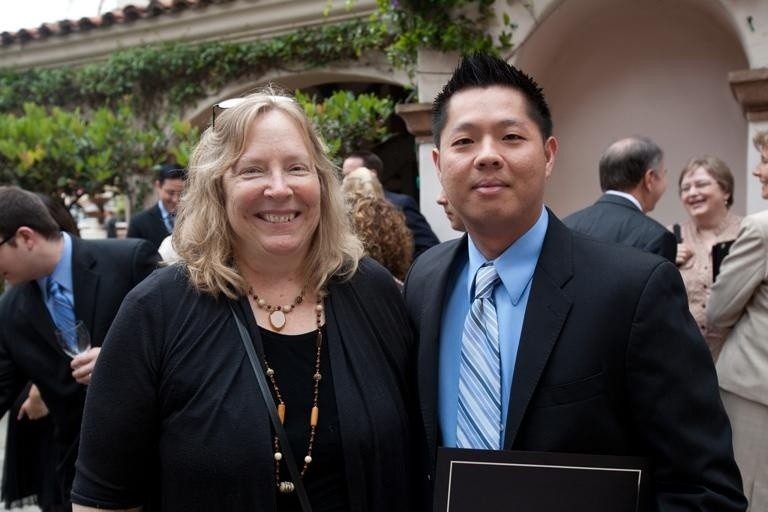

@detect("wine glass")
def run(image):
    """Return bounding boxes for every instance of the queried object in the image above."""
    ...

[54,321,92,358]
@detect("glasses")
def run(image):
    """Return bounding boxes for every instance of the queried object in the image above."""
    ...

[212,94,296,129]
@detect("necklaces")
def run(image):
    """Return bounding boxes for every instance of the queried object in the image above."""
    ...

[235,264,316,332]
[260,284,323,495]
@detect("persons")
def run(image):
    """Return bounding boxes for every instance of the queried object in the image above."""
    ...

[402,47,748,511]
[70,88,433,511]
[338,167,415,294]
[437,174,467,233]
[0,185,170,511]
[663,154,743,338]
[703,126,768,512]
[36,185,116,239]
[339,150,443,259]
[127,162,190,268]
[559,135,678,266]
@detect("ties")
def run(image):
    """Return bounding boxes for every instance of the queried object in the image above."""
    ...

[456,264,502,450]
[50,282,78,354]
[167,214,175,230]
